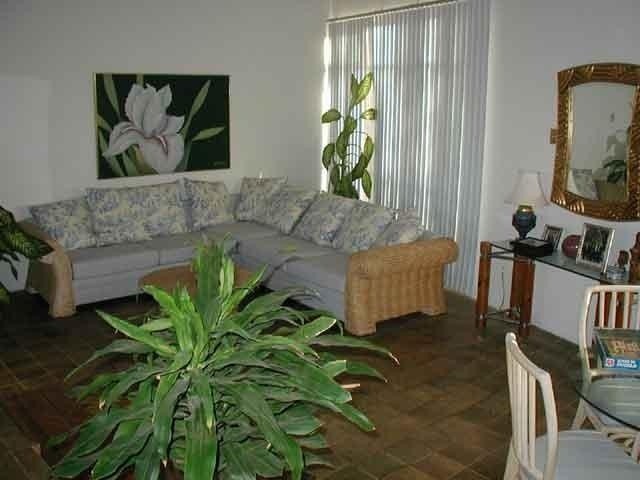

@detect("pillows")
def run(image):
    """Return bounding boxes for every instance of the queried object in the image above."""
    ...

[237,176,420,253]
[29,178,232,253]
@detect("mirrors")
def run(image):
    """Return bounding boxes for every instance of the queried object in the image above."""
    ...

[548,62,640,222]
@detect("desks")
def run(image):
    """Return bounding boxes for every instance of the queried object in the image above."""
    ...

[569,343,640,431]
[473,237,640,338]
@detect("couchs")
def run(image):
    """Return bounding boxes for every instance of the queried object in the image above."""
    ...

[15,176,459,337]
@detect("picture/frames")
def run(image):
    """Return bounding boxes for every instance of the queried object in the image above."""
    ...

[542,224,563,249]
[576,222,616,268]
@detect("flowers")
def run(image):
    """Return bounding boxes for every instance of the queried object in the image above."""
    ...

[96,74,226,175]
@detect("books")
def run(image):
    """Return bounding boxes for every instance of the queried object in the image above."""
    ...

[592,326,639,369]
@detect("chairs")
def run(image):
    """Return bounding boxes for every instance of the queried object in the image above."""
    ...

[502,333,640,480]
[568,285,640,460]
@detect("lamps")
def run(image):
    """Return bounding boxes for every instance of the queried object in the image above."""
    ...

[504,170,549,246]
